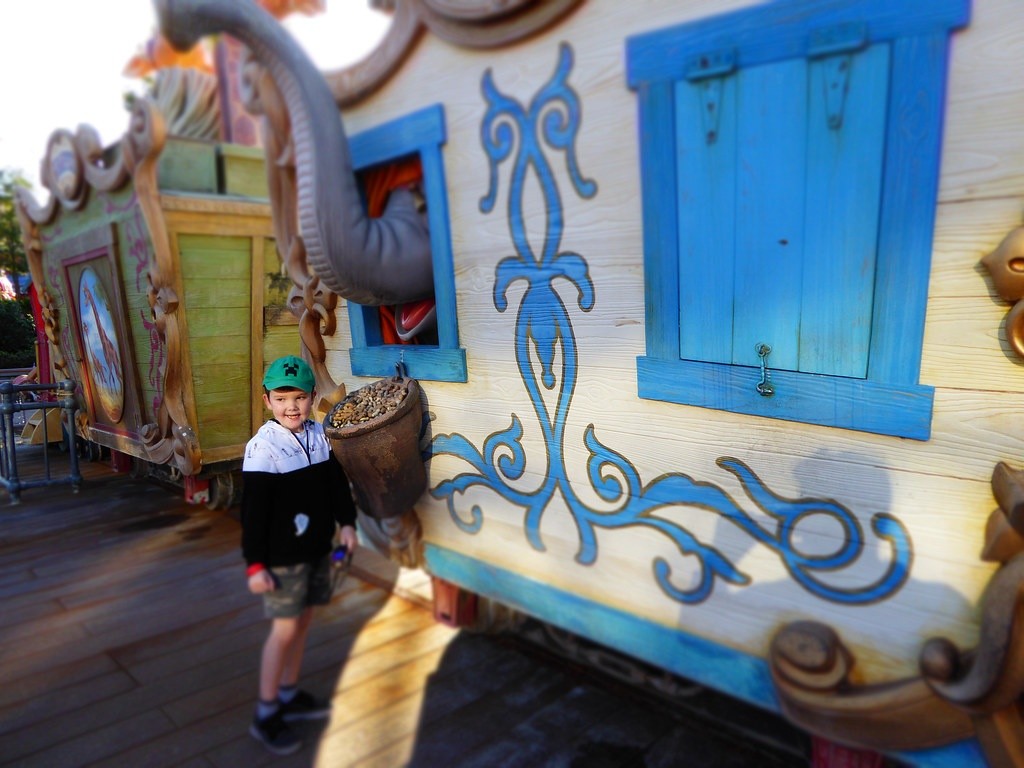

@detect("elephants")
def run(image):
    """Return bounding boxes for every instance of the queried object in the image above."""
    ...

[150,1,439,343]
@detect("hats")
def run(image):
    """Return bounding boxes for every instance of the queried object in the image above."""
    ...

[262,355,316,393]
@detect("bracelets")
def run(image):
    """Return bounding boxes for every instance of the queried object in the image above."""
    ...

[246,563,265,578]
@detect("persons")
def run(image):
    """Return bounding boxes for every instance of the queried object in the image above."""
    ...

[238,352,357,758]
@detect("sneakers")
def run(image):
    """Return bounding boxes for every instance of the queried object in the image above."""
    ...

[275,687,330,720]
[249,707,303,756]
